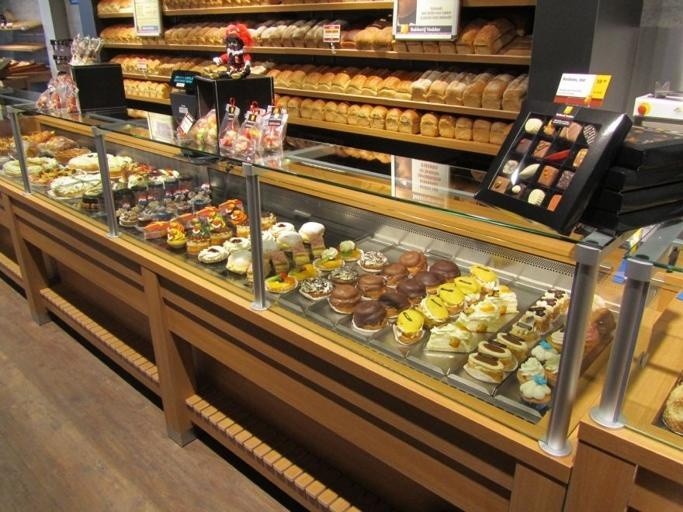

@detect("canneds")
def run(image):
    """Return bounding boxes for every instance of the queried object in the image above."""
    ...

[81,174,193,212]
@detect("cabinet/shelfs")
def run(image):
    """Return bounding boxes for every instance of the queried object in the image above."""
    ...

[94,1,535,198]
[563,221,682,511]
[1,18,51,123]
[0,95,654,511]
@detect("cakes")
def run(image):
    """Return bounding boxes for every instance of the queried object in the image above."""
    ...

[116,189,362,296]
[0,130,181,197]
[393,264,615,410]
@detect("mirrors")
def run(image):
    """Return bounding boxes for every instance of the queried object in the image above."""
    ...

[0,1,54,105]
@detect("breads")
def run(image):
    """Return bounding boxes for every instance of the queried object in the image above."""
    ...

[95,0,529,146]
[490,117,599,212]
[661,386,683,434]
[298,251,461,330]
